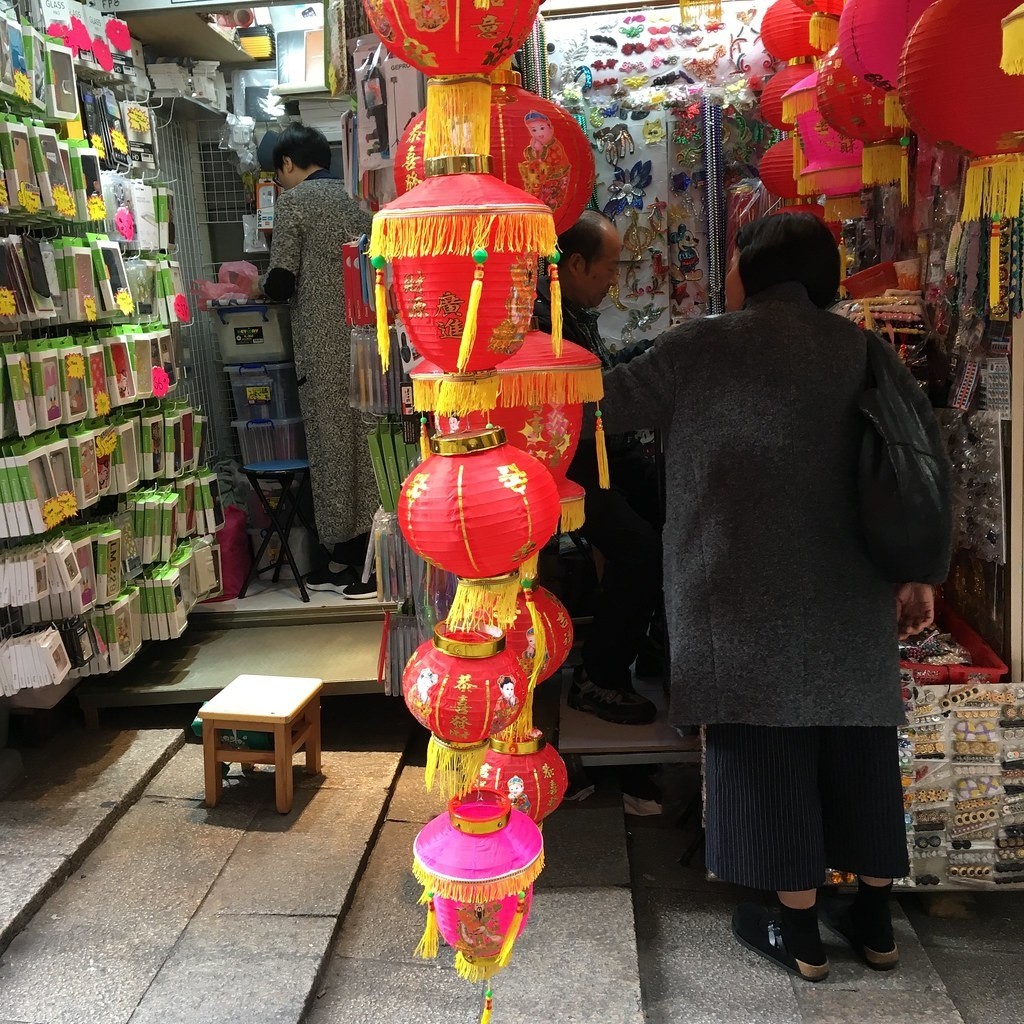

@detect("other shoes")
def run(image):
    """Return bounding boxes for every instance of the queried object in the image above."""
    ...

[732,901,830,982]
[821,897,899,972]
[567,663,657,725]
[306,566,359,594]
[343,572,377,599]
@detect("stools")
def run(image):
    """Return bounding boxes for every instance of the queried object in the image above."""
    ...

[197,674,324,814]
[238,459,324,602]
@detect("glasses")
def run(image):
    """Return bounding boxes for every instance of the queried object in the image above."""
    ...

[272,170,283,188]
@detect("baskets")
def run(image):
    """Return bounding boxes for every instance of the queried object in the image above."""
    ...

[901,599,1008,699]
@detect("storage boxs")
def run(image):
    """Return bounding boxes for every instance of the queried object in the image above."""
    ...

[230,416,305,462]
[222,362,301,419]
[208,300,295,364]
[899,602,1009,686]
[248,480,300,527]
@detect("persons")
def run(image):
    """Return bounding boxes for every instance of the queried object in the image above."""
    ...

[529,207,672,727]
[257,119,379,604]
[571,210,957,985]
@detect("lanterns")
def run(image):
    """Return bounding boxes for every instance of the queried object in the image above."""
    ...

[472,577,575,737]
[400,617,529,798]
[406,314,612,537]
[396,428,565,629]
[359,1,541,160]
[364,154,566,417]
[412,787,545,1024]
[747,1,1024,308]
[474,725,568,832]
[392,58,596,236]
